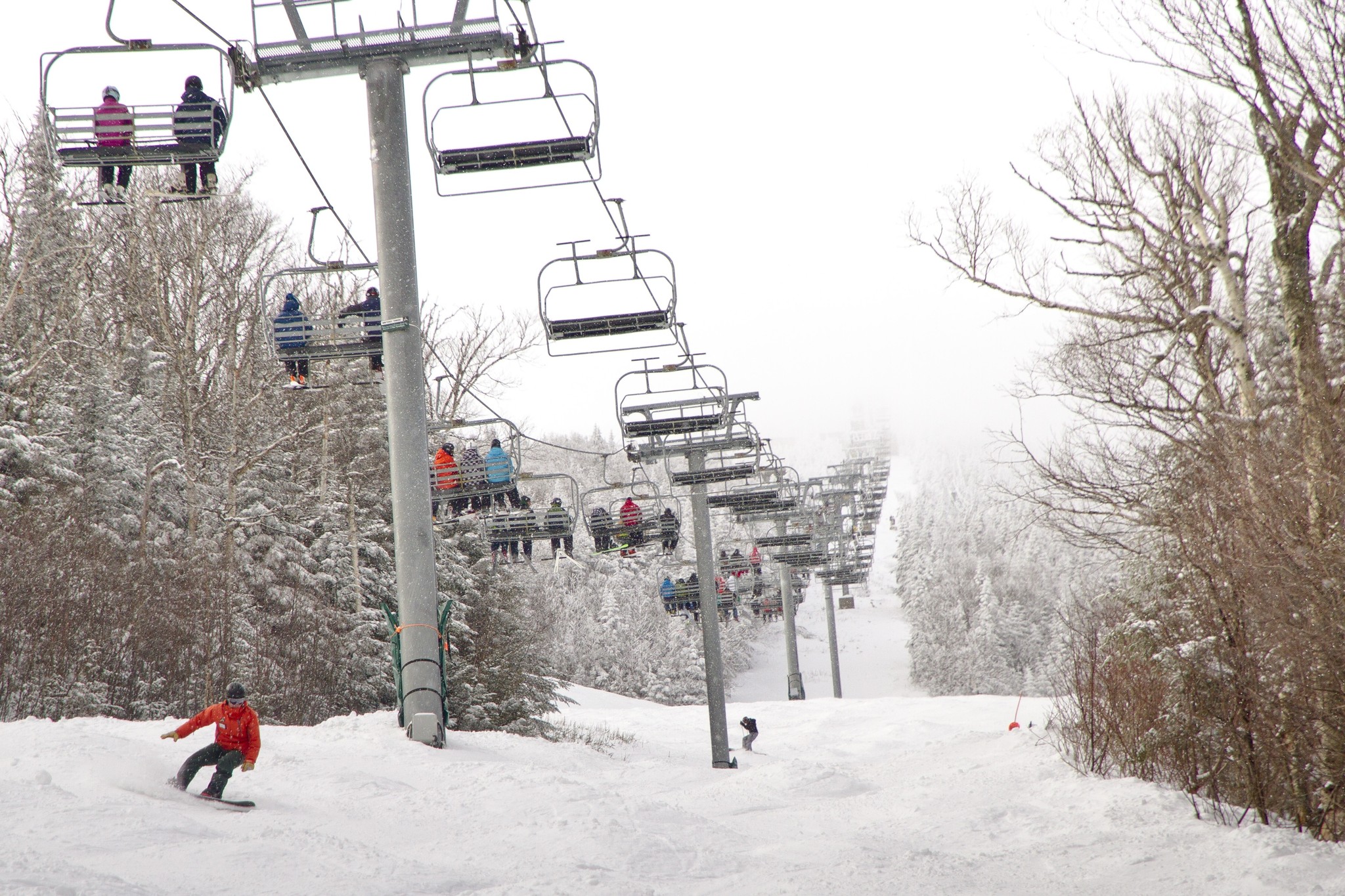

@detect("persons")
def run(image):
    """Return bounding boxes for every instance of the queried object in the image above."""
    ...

[93,86,135,199]
[740,716,758,752]
[273,293,313,387]
[160,682,261,799]
[429,439,809,623]
[173,76,227,194]
[337,288,383,371]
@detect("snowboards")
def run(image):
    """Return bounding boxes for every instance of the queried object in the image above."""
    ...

[166,782,256,808]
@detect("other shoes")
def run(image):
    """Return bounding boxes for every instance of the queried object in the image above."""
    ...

[491,549,574,564]
[103,184,116,198]
[595,542,674,557]
[187,191,197,201]
[200,187,216,195]
[693,618,699,622]
[434,503,520,522]
[115,188,125,200]
[168,778,187,790]
[299,376,307,386]
[291,376,299,383]
[726,616,739,623]
[372,364,383,373]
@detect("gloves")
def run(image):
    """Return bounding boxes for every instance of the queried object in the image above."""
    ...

[241,763,254,772]
[337,316,346,328]
[160,731,179,742]
[740,721,744,725]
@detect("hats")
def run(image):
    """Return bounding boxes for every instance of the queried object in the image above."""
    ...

[282,294,298,307]
[521,496,530,502]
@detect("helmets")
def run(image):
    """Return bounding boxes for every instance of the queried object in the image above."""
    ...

[743,717,748,723]
[466,442,478,451]
[185,76,203,90]
[550,498,562,506]
[102,86,120,102]
[225,681,245,703]
[366,288,379,298]
[442,443,455,456]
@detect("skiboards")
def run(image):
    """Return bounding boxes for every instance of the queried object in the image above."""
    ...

[433,503,520,525]
[588,543,673,559]
[96,188,136,215]
[490,546,585,576]
[728,748,767,755]
[146,188,240,204]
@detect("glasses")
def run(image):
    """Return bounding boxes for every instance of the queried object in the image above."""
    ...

[229,701,242,705]
